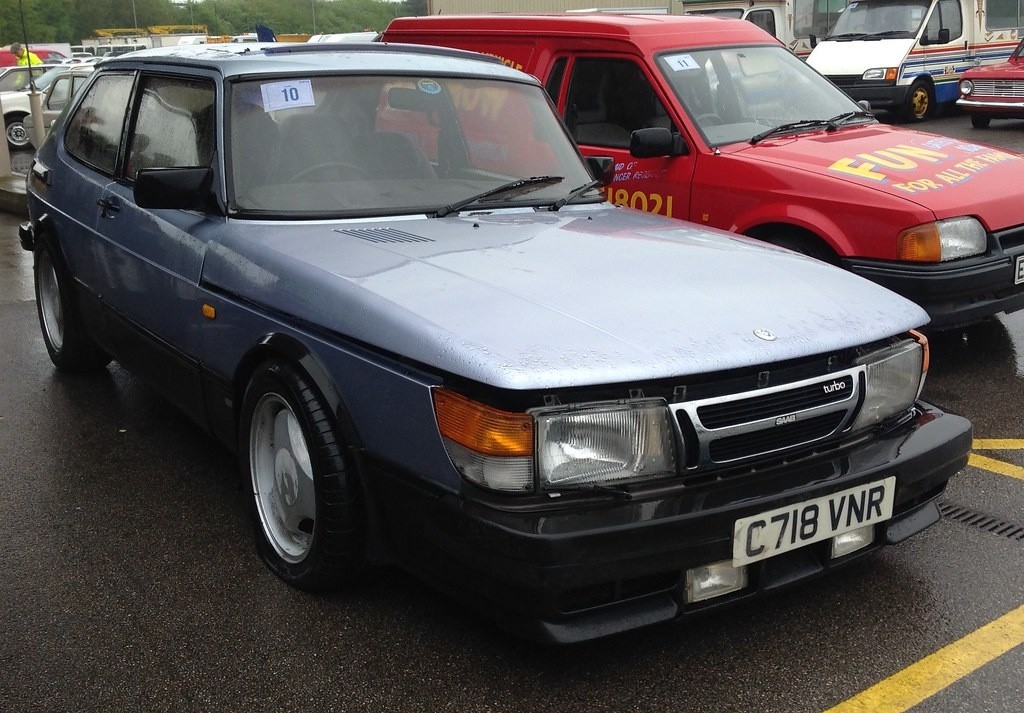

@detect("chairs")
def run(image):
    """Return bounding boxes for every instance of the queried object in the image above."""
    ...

[880,11,904,30]
[577,87,632,147]
[340,131,440,180]
[642,85,672,132]
[196,102,283,184]
[282,113,353,183]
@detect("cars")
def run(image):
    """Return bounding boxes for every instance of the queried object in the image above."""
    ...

[0,23,378,149]
[955,38,1023,130]
[19,41,973,648]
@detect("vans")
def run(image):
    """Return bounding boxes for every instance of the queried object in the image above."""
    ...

[375,11,1023,331]
[682,1,856,117]
[806,0,1024,123]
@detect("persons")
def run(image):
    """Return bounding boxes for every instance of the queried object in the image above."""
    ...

[10,42,43,91]
